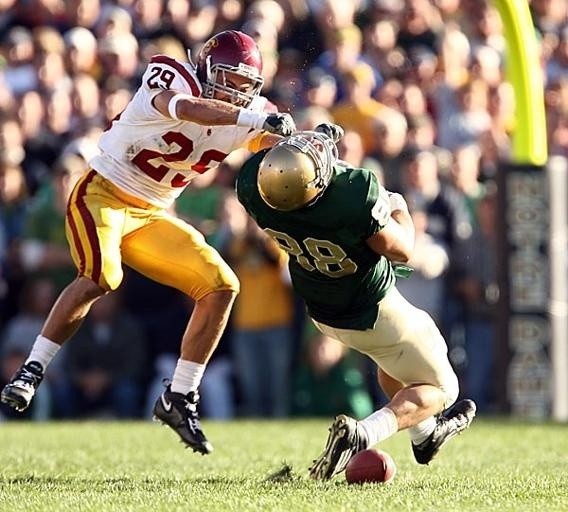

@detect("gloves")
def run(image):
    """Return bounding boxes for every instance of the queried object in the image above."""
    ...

[384,190,411,215]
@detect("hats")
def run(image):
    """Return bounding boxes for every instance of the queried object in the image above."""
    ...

[0,0,142,99]
[241,2,506,166]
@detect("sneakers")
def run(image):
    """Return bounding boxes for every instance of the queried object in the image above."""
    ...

[410,397,478,465]
[1,360,45,414]
[262,111,297,136]
[308,413,371,483]
[151,375,214,455]
[313,122,345,144]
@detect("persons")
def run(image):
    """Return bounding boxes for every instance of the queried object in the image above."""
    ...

[1,31,296,454]
[235,123,477,483]
[1,2,568,422]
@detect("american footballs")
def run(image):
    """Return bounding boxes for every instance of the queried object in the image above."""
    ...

[345,450,392,483]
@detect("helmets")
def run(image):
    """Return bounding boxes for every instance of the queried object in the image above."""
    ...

[256,130,339,213]
[194,28,265,111]
